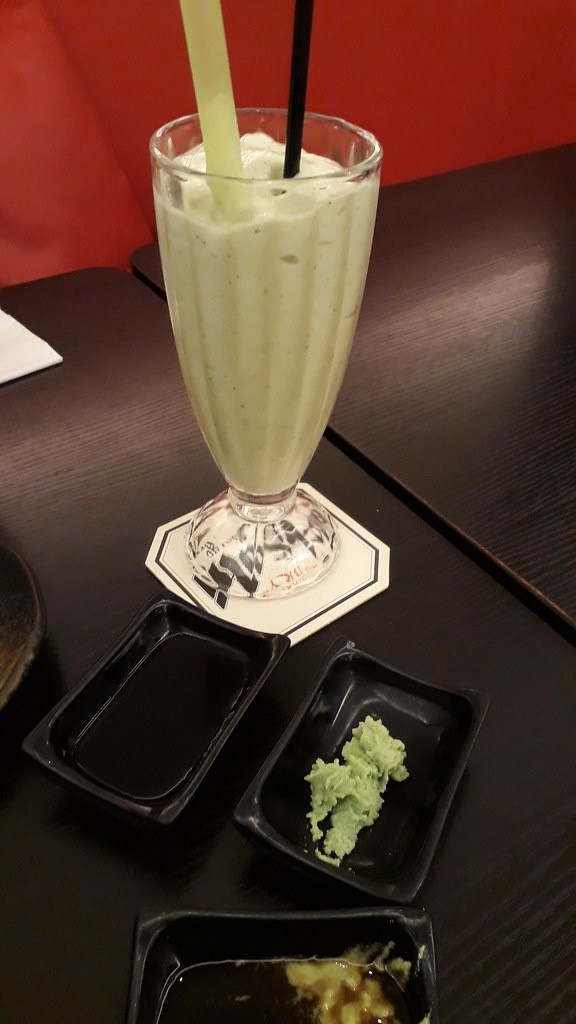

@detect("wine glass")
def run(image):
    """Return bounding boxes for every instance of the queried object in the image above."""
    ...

[152,106,384,603]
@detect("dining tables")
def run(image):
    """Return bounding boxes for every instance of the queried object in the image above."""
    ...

[1,265,576,1023]
[124,142,574,629]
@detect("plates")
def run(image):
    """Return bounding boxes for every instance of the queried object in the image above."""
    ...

[119,901,444,1024]
[22,588,291,824]
[231,633,492,906]
[0,529,44,717]
